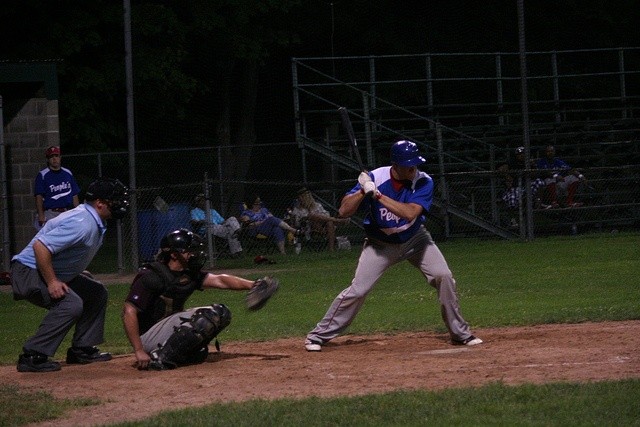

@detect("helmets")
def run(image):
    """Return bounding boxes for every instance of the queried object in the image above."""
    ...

[86,177,130,222]
[390,140,427,166]
[160,228,207,280]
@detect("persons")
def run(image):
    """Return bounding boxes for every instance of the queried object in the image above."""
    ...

[239,193,306,258]
[535,144,587,210]
[188,191,248,259]
[33,144,81,227]
[292,186,352,251]
[122,227,280,372]
[500,146,553,228]
[303,139,485,353]
[7,175,131,373]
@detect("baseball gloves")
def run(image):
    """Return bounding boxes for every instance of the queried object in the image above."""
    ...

[245,276,279,312]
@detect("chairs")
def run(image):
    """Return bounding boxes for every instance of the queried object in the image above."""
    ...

[242,203,276,255]
[290,199,329,252]
[188,219,229,259]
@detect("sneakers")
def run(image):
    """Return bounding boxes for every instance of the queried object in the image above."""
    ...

[17,354,61,372]
[66,346,112,364]
[304,337,322,351]
[552,201,560,208]
[508,217,519,226]
[566,202,580,207]
[536,203,552,210]
[452,335,483,345]
[294,230,305,237]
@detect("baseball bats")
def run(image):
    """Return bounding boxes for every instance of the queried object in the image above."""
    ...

[337,107,374,197]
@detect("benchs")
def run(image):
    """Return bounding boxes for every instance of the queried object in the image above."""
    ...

[465,175,640,230]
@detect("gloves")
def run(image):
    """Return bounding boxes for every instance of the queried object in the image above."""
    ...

[364,181,382,200]
[357,171,372,195]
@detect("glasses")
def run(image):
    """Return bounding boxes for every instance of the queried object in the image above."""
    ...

[517,149,526,153]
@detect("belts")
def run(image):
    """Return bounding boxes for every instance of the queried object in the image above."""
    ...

[46,208,69,212]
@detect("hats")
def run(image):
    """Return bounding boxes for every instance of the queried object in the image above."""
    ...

[296,187,310,194]
[247,193,263,205]
[515,147,525,155]
[48,147,60,156]
